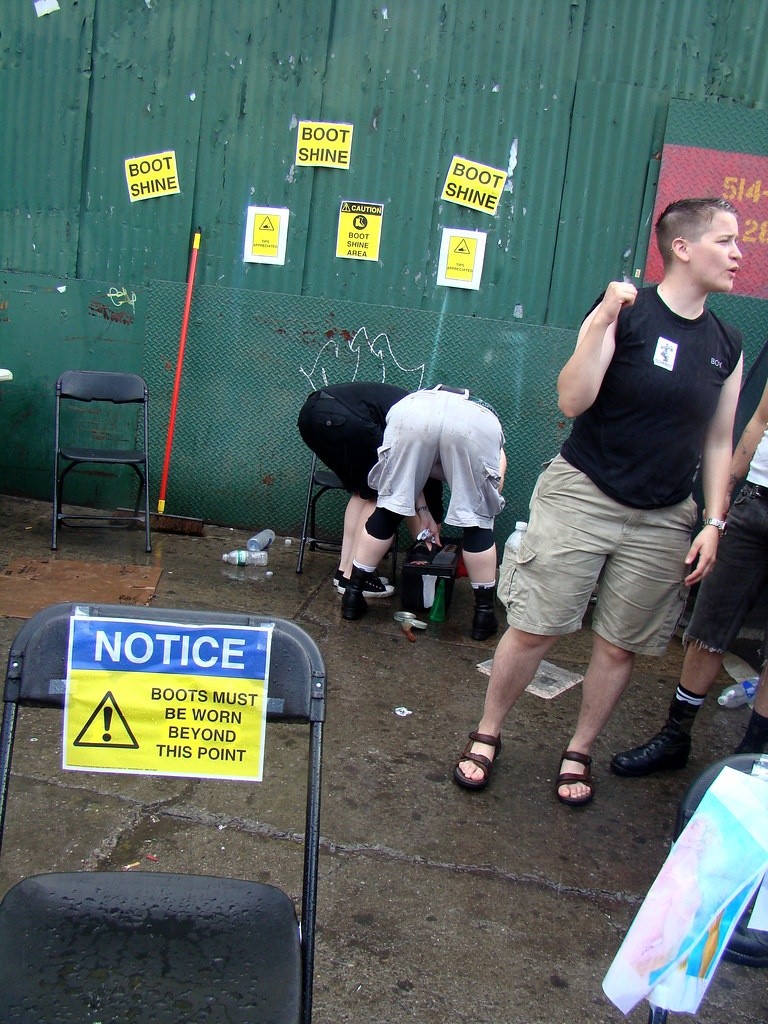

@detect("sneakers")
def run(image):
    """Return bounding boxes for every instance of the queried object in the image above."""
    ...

[332,570,395,598]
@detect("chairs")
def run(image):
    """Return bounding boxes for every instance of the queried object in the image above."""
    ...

[295,451,399,585]
[52,368,152,554]
[602,752,768,1024]
[0,600,330,1024]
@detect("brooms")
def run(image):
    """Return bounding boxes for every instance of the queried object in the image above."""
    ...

[115,226,206,539]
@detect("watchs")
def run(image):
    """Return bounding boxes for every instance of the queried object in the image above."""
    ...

[702,518,729,541]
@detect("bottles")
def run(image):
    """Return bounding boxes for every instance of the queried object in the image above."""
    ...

[222,529,276,566]
[429,579,445,622]
[718,676,761,708]
[506,521,527,550]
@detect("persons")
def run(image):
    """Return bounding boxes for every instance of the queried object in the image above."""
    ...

[299,383,443,599]
[613,344,767,777]
[340,383,508,638]
[452,196,745,803]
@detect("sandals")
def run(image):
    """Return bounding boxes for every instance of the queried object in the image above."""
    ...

[454,729,502,791]
[556,745,595,806]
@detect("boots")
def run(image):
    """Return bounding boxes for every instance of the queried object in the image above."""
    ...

[733,708,767,754]
[469,581,499,641]
[609,693,695,777]
[342,558,378,620]
